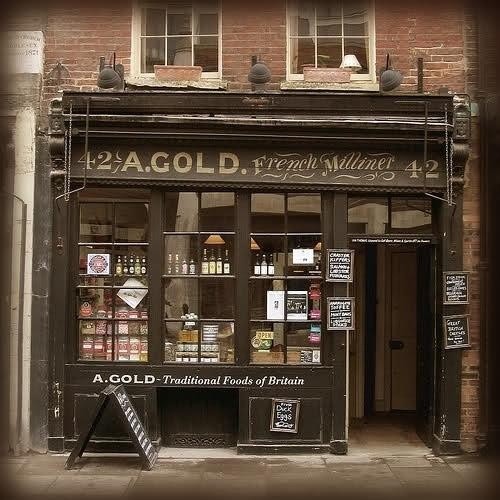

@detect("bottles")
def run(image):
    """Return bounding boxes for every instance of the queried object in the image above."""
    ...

[201,248,232,275]
[115,255,147,276]
[167,254,196,275]
[253,253,275,276]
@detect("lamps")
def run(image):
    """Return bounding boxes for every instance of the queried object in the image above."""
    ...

[97,69,121,89]
[339,53,363,75]
[380,69,403,92]
[248,63,272,84]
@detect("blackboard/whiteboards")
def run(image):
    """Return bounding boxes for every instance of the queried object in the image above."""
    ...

[327,297,356,330]
[270,399,301,434]
[326,248,355,283]
[442,314,472,350]
[442,271,471,305]
[109,384,158,471]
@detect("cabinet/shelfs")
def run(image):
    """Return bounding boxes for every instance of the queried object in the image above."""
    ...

[77,273,324,365]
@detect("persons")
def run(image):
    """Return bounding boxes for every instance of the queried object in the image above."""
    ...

[287,299,306,314]
